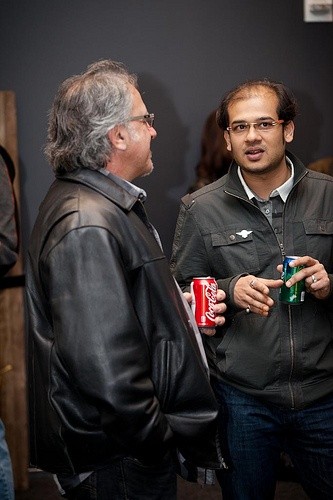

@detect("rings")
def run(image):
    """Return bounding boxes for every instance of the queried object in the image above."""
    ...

[312,275,317,282]
[250,280,255,288]
[246,305,250,312]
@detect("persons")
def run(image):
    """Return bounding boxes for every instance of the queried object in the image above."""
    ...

[186,107,232,195]
[308,156,333,178]
[170,80,333,500]
[0,145,20,500]
[24,59,226,500]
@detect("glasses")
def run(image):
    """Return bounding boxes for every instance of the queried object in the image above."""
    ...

[227,120,289,132]
[122,112,155,127]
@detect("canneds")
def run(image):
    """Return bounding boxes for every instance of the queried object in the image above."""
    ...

[279,256,307,305]
[190,276,218,329]
